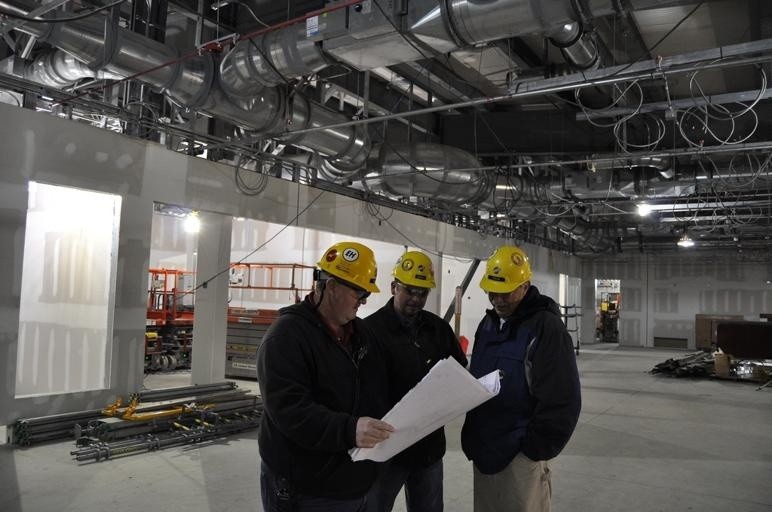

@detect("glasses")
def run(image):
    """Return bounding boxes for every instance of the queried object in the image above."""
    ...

[395,281,432,296]
[336,281,371,301]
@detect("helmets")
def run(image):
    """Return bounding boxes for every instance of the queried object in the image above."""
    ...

[390,250,437,289]
[479,246,533,294]
[317,241,381,294]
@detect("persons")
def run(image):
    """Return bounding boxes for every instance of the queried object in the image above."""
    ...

[363,249,468,511]
[459,245,583,511]
[254,242,395,512]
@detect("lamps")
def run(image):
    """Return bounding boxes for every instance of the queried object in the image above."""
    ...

[677,233,696,248]
